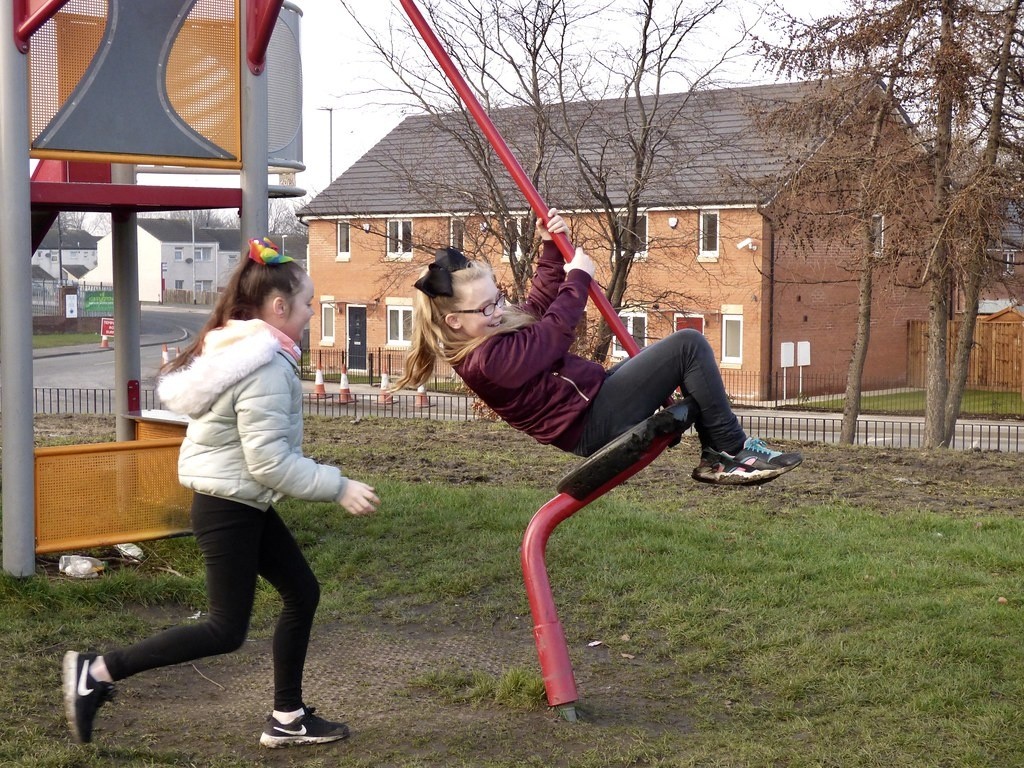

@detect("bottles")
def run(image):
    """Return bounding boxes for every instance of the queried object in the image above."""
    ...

[60,556,107,575]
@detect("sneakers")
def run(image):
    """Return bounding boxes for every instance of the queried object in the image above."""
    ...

[260,705,350,748]
[693,436,802,485]
[62,651,118,745]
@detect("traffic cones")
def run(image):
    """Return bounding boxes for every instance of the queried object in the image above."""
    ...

[373,366,398,405]
[176,346,181,358]
[307,362,332,399]
[333,363,358,404]
[162,343,171,365]
[99,335,112,348]
[409,381,436,408]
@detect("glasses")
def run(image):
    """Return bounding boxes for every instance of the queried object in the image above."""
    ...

[451,291,506,317]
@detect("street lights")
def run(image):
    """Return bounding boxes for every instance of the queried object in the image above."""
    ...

[281,234,288,256]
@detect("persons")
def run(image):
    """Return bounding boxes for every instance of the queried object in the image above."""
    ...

[379,208,801,487]
[60,234,380,748]
